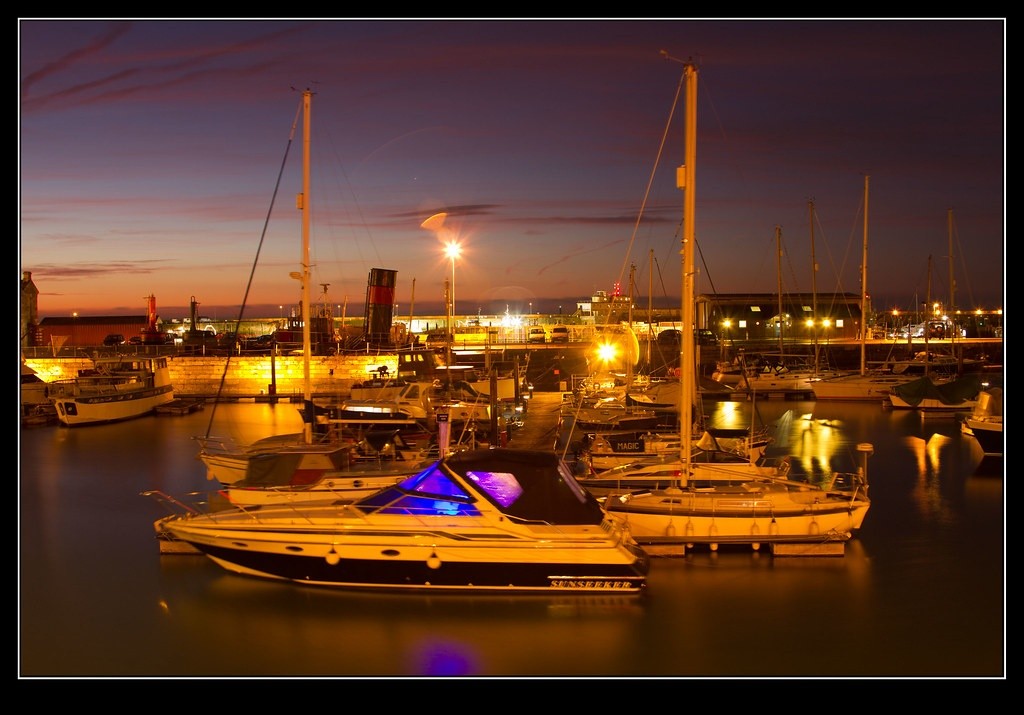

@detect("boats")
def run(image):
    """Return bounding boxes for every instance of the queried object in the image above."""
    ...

[21,359,55,426]
[151,449,653,595]
[46,356,175,428]
[153,400,205,416]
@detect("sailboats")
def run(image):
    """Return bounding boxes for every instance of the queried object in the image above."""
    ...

[557,46,874,562]
[191,85,456,490]
[298,166,1006,472]
